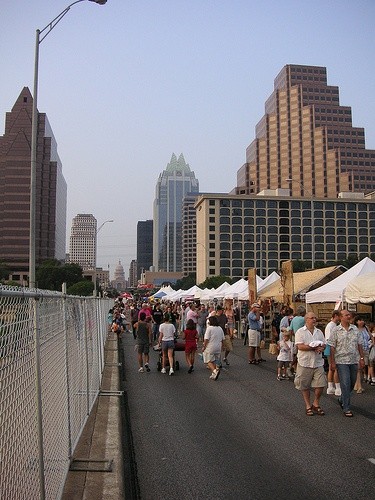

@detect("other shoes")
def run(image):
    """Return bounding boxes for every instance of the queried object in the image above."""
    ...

[363,377,367,382]
[354,385,365,393]
[335,389,342,395]
[326,387,335,394]
[287,370,293,377]
[144,363,151,372]
[169,368,174,376]
[126,330,132,334]
[188,365,193,374]
[161,369,166,374]
[277,376,281,380]
[209,368,220,381]
[366,378,375,385]
[281,375,289,380]
[137,368,144,372]
[222,357,230,365]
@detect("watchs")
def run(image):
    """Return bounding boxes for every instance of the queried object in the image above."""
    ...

[360,357,364,359]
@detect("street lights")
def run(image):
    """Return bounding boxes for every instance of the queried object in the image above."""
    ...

[28,0,108,319]
[92,219,113,297]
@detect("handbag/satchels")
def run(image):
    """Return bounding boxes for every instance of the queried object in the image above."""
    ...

[368,339,373,348]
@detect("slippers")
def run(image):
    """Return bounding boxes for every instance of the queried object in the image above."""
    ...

[338,397,344,408]
[344,410,353,417]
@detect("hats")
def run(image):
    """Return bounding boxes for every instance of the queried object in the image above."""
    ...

[251,303,260,309]
[215,305,224,312]
[142,303,147,306]
[309,341,324,348]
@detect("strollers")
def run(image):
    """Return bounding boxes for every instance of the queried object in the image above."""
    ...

[156,336,180,372]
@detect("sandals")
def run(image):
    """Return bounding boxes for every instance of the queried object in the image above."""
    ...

[257,358,266,362]
[248,358,259,364]
[305,406,314,416]
[311,404,325,416]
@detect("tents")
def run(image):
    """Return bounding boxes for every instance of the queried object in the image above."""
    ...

[150,257,375,313]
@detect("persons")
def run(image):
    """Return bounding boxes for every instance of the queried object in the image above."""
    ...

[324,309,375,418]
[182,319,199,373]
[202,316,226,380]
[294,311,328,416]
[108,297,306,417]
[133,312,153,373]
